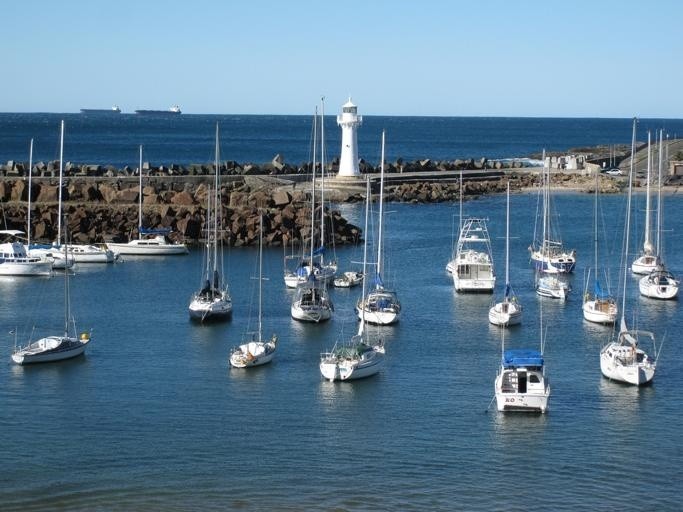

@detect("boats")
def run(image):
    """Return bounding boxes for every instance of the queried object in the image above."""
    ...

[494,349,551,414]
[135,105,181,116]
[450,217,497,294]
[0,230,55,276]
[79,106,120,113]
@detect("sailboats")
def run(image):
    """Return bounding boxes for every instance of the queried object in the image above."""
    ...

[24,139,77,270]
[188,121,233,321]
[11,227,90,364]
[533,118,681,386]
[231,214,278,370]
[445,172,463,274]
[488,181,524,326]
[105,144,190,256]
[53,119,120,264]
[285,97,401,384]
